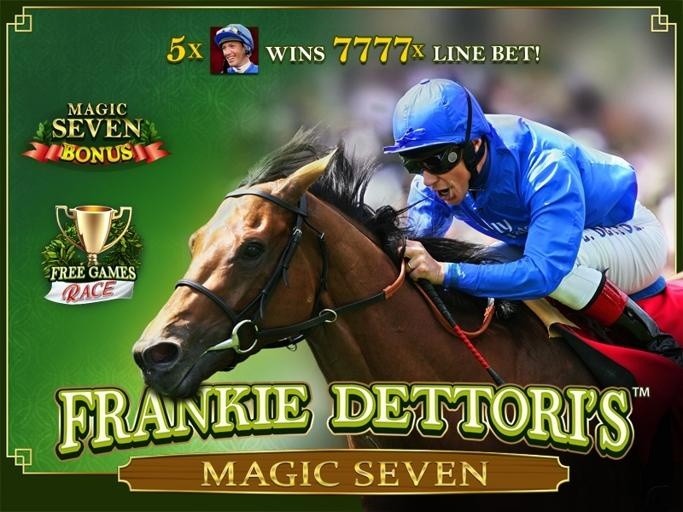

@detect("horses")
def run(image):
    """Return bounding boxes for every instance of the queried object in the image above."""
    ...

[131,119,683,512]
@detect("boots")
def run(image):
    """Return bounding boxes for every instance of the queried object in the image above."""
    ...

[578,271,682,367]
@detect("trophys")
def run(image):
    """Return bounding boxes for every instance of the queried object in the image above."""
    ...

[55,204,132,268]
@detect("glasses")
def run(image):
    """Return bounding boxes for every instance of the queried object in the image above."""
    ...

[215,27,247,41]
[401,134,469,175]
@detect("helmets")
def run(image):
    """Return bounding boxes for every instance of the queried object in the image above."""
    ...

[383,77,490,154]
[214,23,253,53]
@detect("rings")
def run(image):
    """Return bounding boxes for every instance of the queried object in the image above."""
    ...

[407,262,414,270]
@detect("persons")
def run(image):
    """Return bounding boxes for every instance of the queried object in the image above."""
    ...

[381,78,682,366]
[215,23,258,74]
